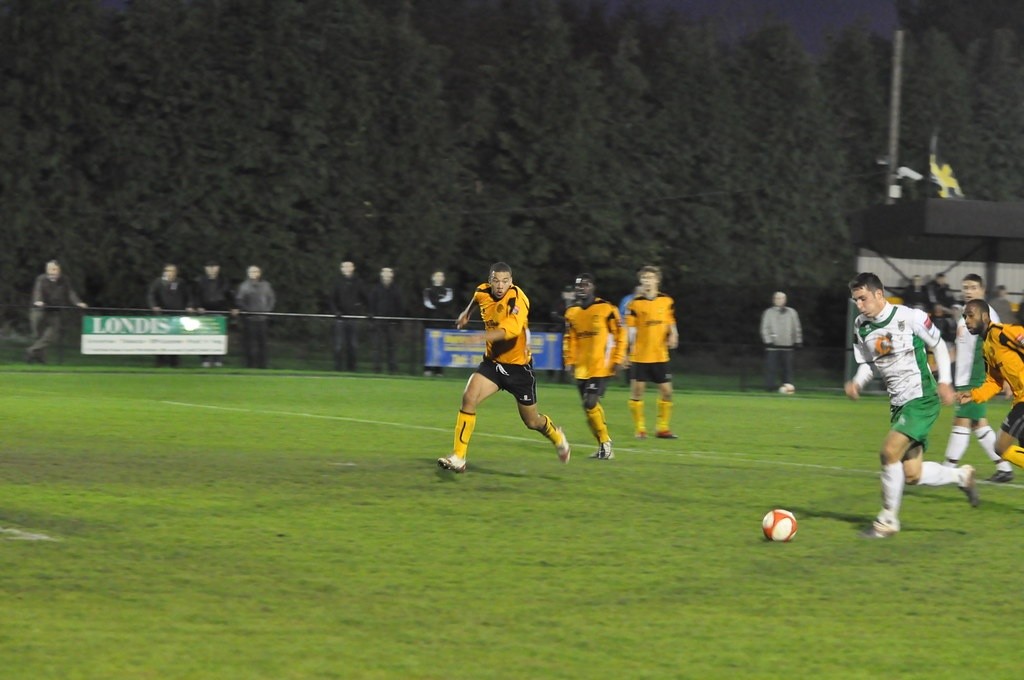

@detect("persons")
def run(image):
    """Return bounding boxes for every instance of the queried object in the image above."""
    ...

[844,273,979,538]
[329,263,369,373]
[437,262,572,474]
[955,298,1024,470]
[904,271,1024,345]
[760,292,803,395]
[149,265,194,369]
[195,262,237,369]
[619,264,679,437]
[370,265,406,374]
[24,261,88,365]
[423,272,452,377]
[941,274,1015,484]
[552,277,627,460]
[237,266,275,368]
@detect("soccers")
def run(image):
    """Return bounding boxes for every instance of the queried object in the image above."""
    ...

[779,383,795,394]
[762,509,799,542]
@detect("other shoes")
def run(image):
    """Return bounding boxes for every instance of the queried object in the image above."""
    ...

[214,361,223,368]
[201,361,211,369]
[423,370,433,378]
[23,352,46,365]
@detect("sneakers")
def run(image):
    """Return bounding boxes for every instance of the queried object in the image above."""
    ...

[957,464,981,508]
[554,426,571,465]
[437,454,467,474]
[983,467,1014,484]
[854,508,902,541]
[655,430,679,439]
[634,431,649,440]
[588,437,613,460]
